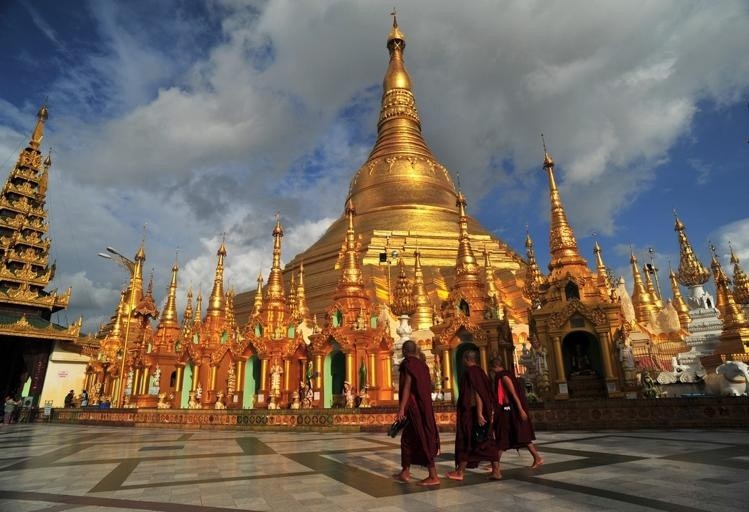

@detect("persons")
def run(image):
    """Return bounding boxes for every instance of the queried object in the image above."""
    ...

[3,395,20,425]
[1,396,10,422]
[79,388,90,408]
[485,357,545,471]
[444,345,503,481]
[390,340,442,487]
[63,389,77,408]
[525,334,550,391]
[98,391,107,411]
[614,328,641,388]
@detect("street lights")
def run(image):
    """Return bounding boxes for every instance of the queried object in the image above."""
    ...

[99,245,137,409]
